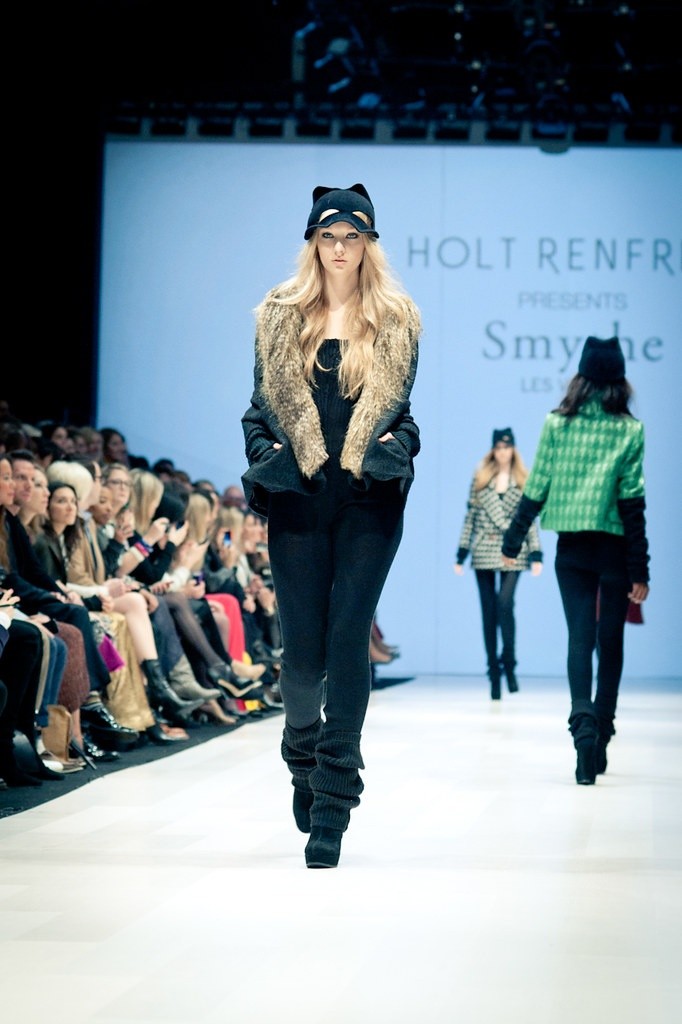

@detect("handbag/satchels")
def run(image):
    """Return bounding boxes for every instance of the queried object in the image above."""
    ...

[37,704,71,763]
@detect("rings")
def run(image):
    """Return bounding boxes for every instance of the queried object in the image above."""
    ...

[119,525,125,530]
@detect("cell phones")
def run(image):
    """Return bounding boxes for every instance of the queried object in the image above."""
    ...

[192,573,202,587]
[223,530,232,549]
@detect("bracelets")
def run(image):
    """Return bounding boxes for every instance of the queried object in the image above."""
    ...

[129,546,145,562]
[140,539,153,552]
[135,542,149,557]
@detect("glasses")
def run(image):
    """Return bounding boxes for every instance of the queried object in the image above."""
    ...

[108,480,132,488]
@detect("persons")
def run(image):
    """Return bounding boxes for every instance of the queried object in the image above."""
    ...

[0,420,400,785]
[503,337,648,783]
[454,428,543,698]
[243,179,422,869]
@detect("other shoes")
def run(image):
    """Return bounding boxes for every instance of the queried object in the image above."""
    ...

[369,640,393,664]
[0,657,282,787]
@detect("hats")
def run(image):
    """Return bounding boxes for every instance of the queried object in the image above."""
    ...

[577,335,625,385]
[304,180,378,240]
[491,427,515,446]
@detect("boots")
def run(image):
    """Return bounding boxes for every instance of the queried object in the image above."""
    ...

[501,654,518,693]
[279,722,322,833]
[569,701,594,786]
[594,699,617,773]
[487,657,503,701]
[304,731,366,865]
